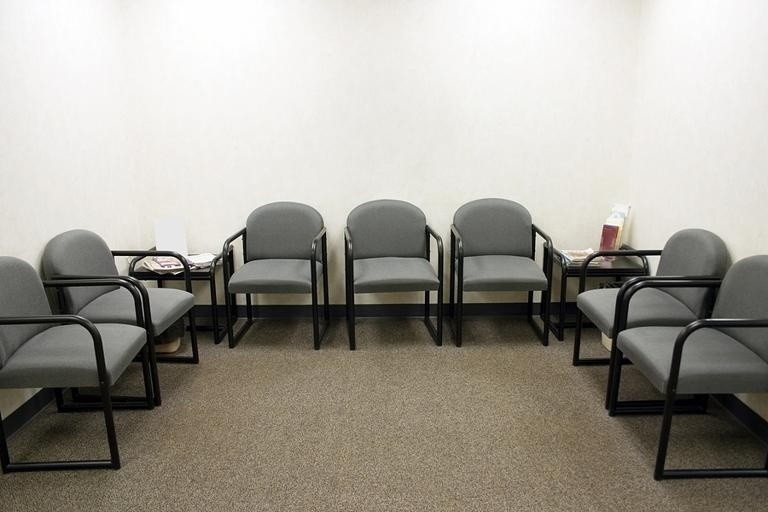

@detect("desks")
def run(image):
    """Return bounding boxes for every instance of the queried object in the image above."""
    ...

[128,246,238,345]
[540,241,649,341]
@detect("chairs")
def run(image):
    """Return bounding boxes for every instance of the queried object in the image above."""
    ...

[0,255,153,471]
[223,202,330,350]
[573,229,729,366]
[344,200,443,351]
[450,198,553,347]
[43,231,199,406]
[608,254,767,481]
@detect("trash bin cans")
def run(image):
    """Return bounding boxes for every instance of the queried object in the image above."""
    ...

[146,316,185,353]
[597,280,625,352]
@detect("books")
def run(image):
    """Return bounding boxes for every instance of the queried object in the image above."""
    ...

[560,245,605,268]
[142,251,220,276]
